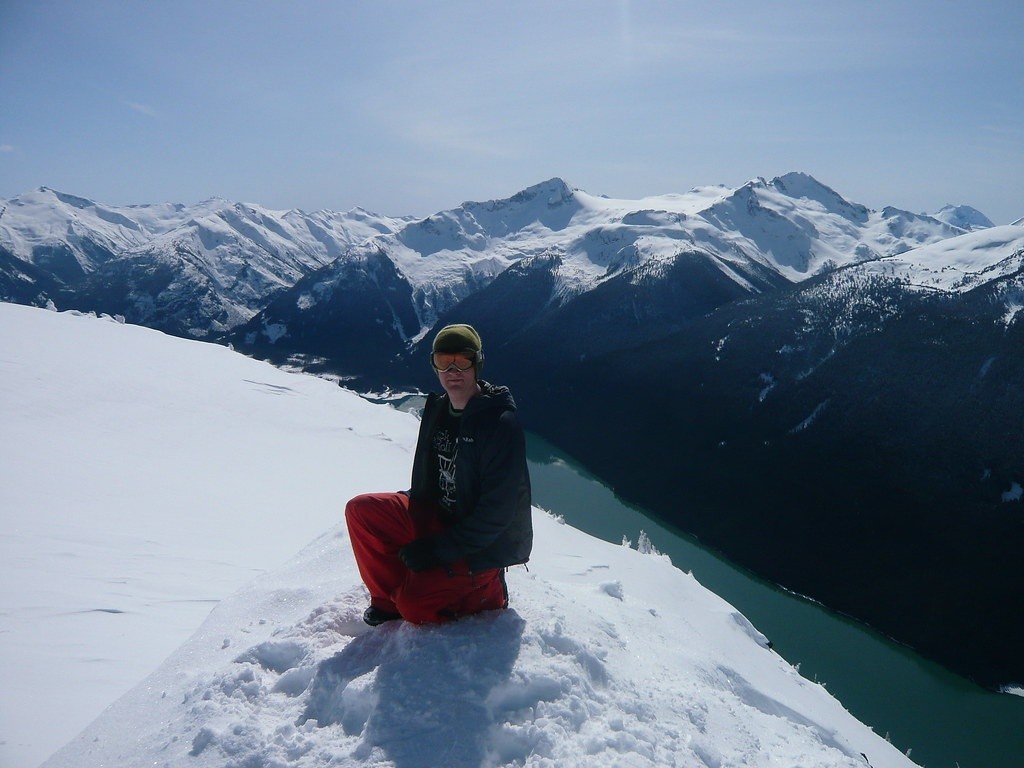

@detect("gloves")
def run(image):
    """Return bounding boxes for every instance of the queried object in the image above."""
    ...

[399,540,435,571]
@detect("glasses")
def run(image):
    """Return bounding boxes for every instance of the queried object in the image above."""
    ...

[430,347,485,376]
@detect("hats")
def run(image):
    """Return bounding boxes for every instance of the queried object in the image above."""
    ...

[431,324,483,352]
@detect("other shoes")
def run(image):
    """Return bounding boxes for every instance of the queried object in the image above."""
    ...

[363,607,401,626]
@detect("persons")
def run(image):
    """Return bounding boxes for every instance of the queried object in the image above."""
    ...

[344,323,534,626]
